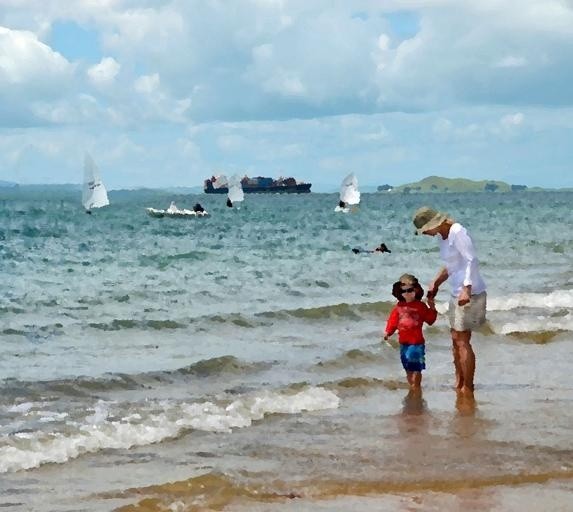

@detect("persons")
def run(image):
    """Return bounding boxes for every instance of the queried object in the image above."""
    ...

[383,207,486,395]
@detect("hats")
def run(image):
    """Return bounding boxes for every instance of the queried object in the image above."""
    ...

[412,207,449,235]
[392,273,424,300]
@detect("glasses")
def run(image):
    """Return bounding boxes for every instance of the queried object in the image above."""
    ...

[401,287,415,293]
